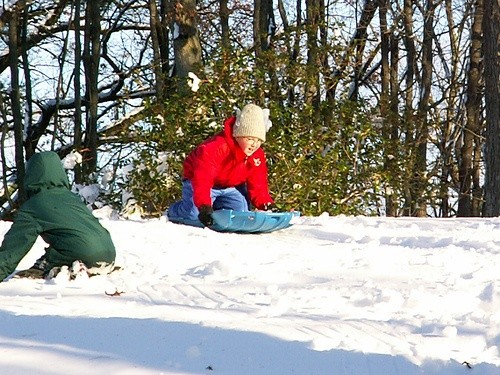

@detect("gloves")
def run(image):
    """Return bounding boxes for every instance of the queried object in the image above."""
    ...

[259,202,281,213]
[198,205,213,226]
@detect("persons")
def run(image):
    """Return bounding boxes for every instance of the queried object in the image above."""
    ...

[167,104,282,228]
[0,150,116,283]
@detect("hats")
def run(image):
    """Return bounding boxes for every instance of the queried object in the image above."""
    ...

[232,104,266,141]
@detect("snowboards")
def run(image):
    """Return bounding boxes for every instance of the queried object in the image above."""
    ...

[163,210,301,234]
